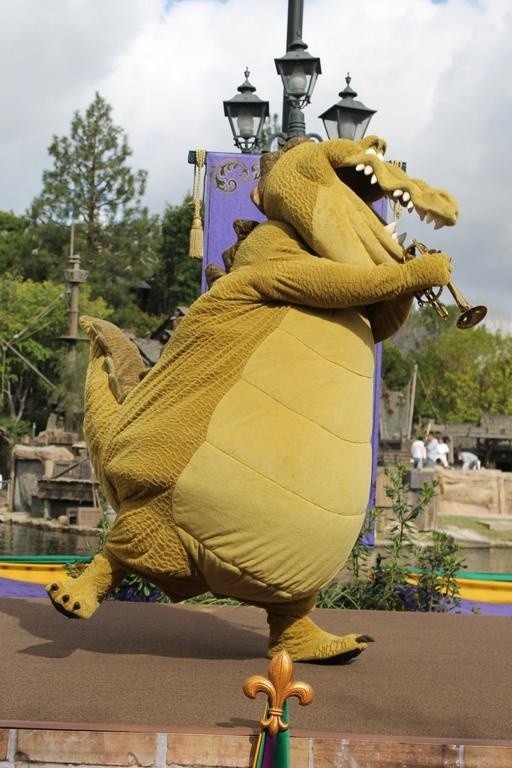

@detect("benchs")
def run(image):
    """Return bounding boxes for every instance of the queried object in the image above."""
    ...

[201,150,391,550]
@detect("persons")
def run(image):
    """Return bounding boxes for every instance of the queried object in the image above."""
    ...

[409,431,482,472]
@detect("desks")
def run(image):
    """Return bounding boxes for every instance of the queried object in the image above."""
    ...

[220,29,379,154]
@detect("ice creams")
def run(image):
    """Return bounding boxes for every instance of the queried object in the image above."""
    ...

[404,239,488,329]
